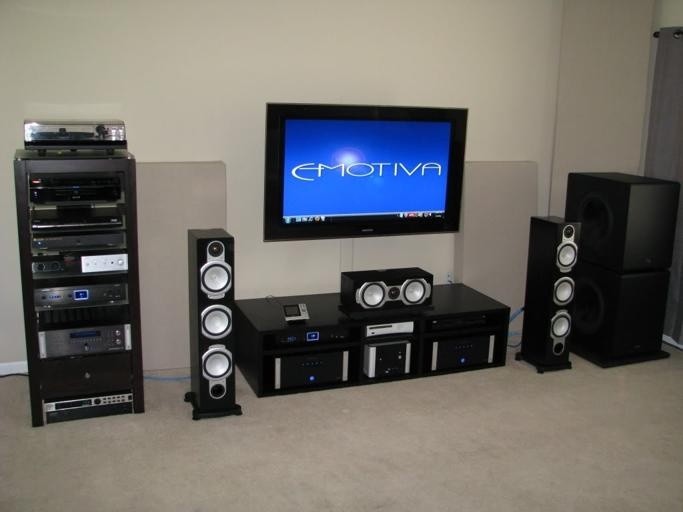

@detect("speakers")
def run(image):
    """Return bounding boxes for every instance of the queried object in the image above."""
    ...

[570,273,671,369]
[515,213,581,376]
[341,265,435,313]
[564,171,681,275]
[186,227,242,420]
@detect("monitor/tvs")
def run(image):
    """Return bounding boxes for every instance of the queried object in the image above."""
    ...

[262,102,470,242]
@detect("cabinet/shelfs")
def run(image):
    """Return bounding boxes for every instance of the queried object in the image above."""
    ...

[235,283,510,399]
